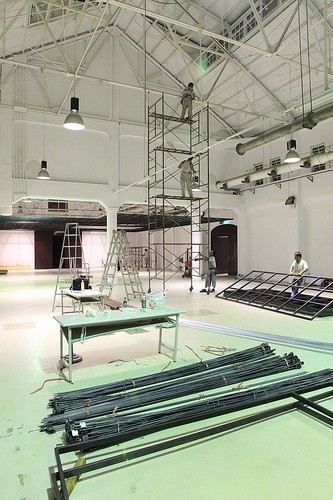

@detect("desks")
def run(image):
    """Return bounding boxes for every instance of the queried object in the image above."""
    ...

[52,304,186,383]
[59,287,107,315]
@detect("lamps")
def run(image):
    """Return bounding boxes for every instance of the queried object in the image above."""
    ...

[285,196,295,206]
[36,68,50,179]
[190,182,200,192]
[284,63,300,163]
[64,0,85,131]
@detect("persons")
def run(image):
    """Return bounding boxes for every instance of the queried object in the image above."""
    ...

[116,253,120,271]
[178,156,197,198]
[179,81,194,119]
[195,250,216,292]
[143,248,148,268]
[289,252,308,300]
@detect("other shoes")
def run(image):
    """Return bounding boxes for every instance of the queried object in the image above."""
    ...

[200,289,206,292]
[211,289,215,292]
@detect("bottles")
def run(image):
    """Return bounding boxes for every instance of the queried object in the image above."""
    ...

[99,294,105,311]
[80,279,84,292]
[123,297,127,307]
[141,296,146,312]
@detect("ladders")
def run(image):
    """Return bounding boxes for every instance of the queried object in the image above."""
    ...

[99,230,146,300]
[52,223,88,314]
